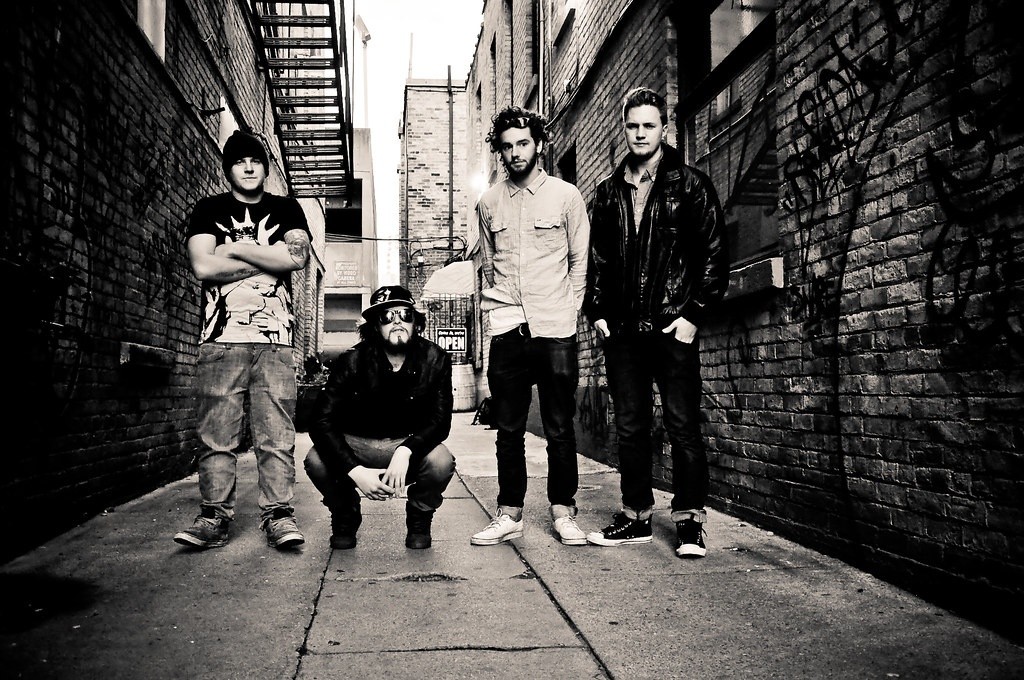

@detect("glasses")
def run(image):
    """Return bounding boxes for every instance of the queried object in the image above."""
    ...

[496,116,532,131]
[376,308,415,325]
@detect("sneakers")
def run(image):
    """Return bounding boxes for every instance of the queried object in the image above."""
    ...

[676,518,707,558]
[470,515,524,545]
[586,511,654,547]
[550,515,588,546]
[259,514,306,548]
[172,513,230,548]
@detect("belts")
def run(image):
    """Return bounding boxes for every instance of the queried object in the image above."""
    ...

[500,323,534,337]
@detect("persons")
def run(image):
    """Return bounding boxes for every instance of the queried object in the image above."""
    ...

[469,105,591,547]
[303,286,456,550]
[583,88,732,558]
[173,130,313,549]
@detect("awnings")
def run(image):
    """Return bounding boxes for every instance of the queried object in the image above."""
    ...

[420,260,475,301]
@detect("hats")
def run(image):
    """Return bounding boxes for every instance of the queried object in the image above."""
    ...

[361,285,416,322]
[222,130,270,182]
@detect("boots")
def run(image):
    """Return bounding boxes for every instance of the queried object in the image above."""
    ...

[330,496,363,549]
[405,502,437,549]
